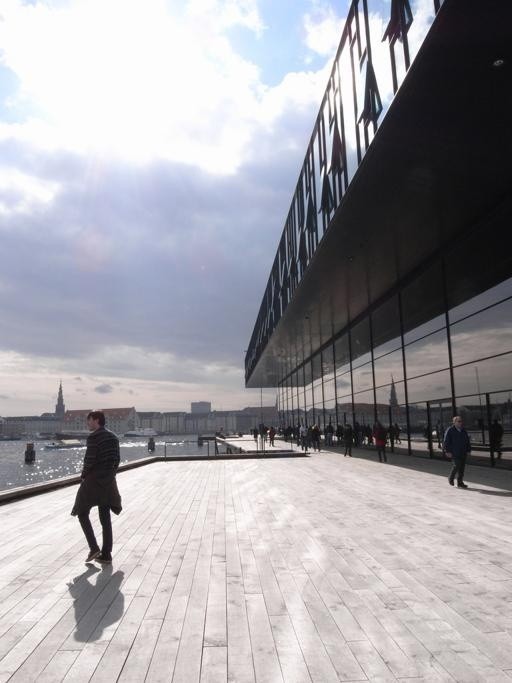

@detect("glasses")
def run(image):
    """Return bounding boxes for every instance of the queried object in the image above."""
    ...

[455,420,464,423]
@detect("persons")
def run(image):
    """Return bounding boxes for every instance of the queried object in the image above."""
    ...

[442,416,471,488]
[71,412,122,563]
[254,419,401,463]
[489,418,504,466]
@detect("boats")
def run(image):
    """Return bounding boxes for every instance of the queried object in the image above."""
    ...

[45,439,81,447]
[148,438,155,454]
[124,428,158,437]
[25,443,35,461]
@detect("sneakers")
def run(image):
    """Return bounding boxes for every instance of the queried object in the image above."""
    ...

[85,547,112,563]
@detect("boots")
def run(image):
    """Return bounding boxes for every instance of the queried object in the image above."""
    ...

[457,471,468,489]
[448,466,456,486]
